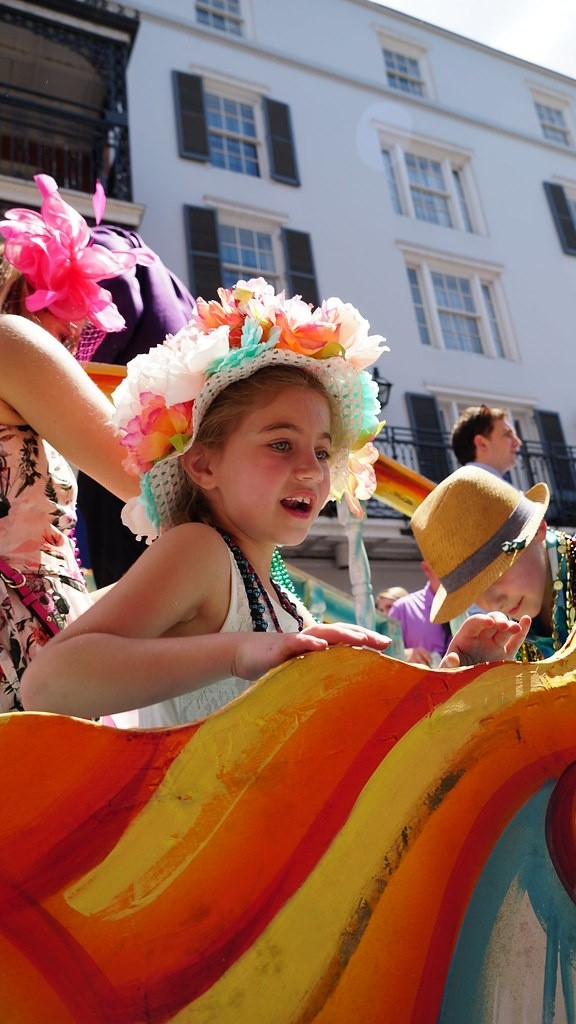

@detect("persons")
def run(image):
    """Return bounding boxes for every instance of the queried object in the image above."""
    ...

[0,244,145,731]
[19,356,530,729]
[372,400,576,668]
[71,224,196,587]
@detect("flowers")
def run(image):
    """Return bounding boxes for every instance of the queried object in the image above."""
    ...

[106,276,389,551]
[0,171,162,336]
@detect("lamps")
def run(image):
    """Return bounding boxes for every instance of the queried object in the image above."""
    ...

[371,365,393,412]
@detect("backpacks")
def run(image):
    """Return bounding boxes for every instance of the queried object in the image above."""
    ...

[88,225,197,366]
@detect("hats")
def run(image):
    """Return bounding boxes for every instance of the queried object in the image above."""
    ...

[0,174,135,332]
[410,466,550,624]
[111,277,386,546]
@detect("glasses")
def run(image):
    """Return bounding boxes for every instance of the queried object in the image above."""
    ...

[479,404,492,420]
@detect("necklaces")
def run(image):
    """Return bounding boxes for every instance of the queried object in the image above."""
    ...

[514,528,576,662]
[205,520,305,634]
